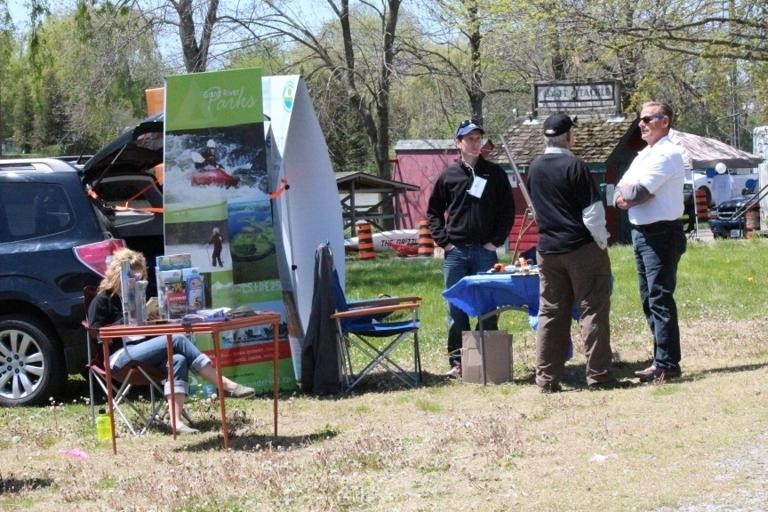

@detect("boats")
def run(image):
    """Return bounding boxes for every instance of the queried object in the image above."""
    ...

[188,169,241,186]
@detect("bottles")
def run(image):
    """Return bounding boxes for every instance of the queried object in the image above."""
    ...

[96,408,113,440]
[186,267,205,312]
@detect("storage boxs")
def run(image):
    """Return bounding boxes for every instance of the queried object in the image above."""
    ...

[458,328,515,389]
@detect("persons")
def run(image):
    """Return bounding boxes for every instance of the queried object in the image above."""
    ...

[525,112,636,395]
[612,100,689,381]
[426,120,517,379]
[204,226,226,268]
[85,248,257,435]
[192,139,231,175]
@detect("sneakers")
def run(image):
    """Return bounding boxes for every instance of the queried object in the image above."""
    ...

[216,382,256,401]
[586,378,641,391]
[541,382,562,393]
[445,364,462,380]
[165,422,200,437]
[633,361,682,382]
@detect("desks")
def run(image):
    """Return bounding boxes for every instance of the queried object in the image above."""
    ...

[94,304,286,458]
[439,263,579,388]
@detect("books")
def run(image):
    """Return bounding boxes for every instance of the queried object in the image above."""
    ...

[155,252,208,324]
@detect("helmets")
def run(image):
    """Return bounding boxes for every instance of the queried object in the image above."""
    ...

[207,139,216,149]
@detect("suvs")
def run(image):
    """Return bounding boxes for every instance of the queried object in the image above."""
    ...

[0,105,167,417]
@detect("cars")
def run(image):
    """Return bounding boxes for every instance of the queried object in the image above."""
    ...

[705,180,760,240]
[692,168,716,208]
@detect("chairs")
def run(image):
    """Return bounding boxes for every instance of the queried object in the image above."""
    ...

[312,240,426,397]
[79,283,200,445]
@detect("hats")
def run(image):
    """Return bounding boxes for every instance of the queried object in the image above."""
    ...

[544,111,579,138]
[454,118,485,138]
